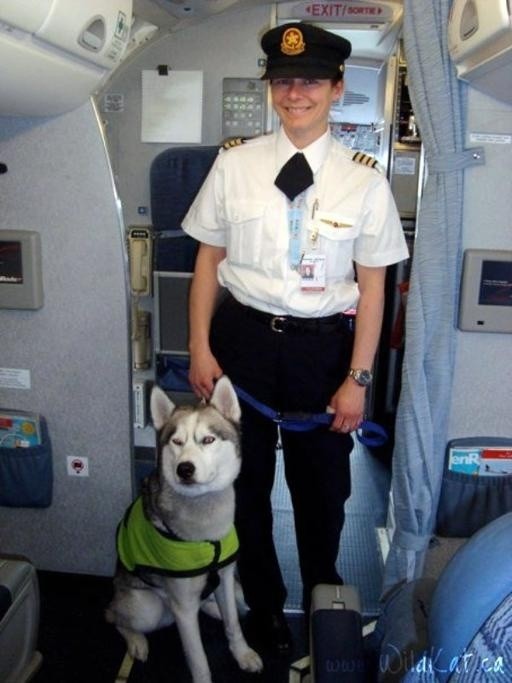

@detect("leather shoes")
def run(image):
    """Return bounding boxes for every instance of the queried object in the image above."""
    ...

[252,607,297,660]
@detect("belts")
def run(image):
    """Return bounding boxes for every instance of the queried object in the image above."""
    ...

[224,289,351,333]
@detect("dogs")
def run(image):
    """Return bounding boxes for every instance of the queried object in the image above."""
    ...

[100,372,262,683]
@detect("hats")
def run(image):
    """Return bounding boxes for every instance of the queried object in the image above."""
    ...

[258,21,353,82]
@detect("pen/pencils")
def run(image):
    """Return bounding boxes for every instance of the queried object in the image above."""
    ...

[311,199,319,220]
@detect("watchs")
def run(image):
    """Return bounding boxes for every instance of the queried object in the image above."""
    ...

[347,366,375,386]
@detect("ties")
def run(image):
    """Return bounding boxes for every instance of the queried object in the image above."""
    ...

[274,152,314,202]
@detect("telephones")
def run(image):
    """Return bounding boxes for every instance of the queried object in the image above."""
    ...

[128,228,152,298]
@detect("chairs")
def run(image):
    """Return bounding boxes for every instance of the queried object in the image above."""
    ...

[0,559,43,683]
[310,508,510,683]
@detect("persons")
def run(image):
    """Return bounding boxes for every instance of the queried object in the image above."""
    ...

[178,20,411,657]
